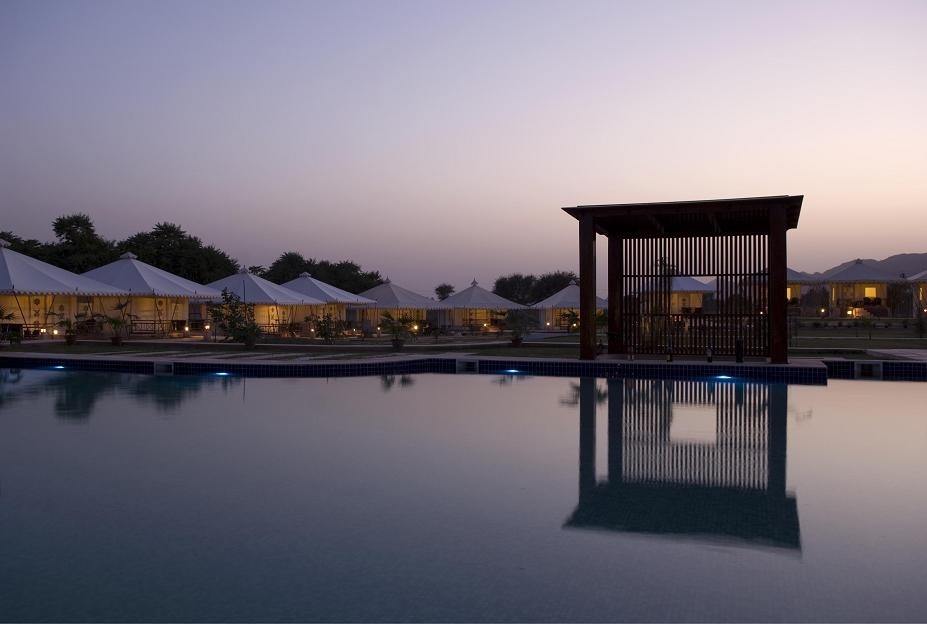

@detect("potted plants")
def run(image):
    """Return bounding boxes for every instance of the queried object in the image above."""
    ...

[58,319,78,345]
[505,309,528,348]
[106,317,126,346]
[380,312,413,353]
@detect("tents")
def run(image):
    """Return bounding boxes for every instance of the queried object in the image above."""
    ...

[0,239,927,338]
